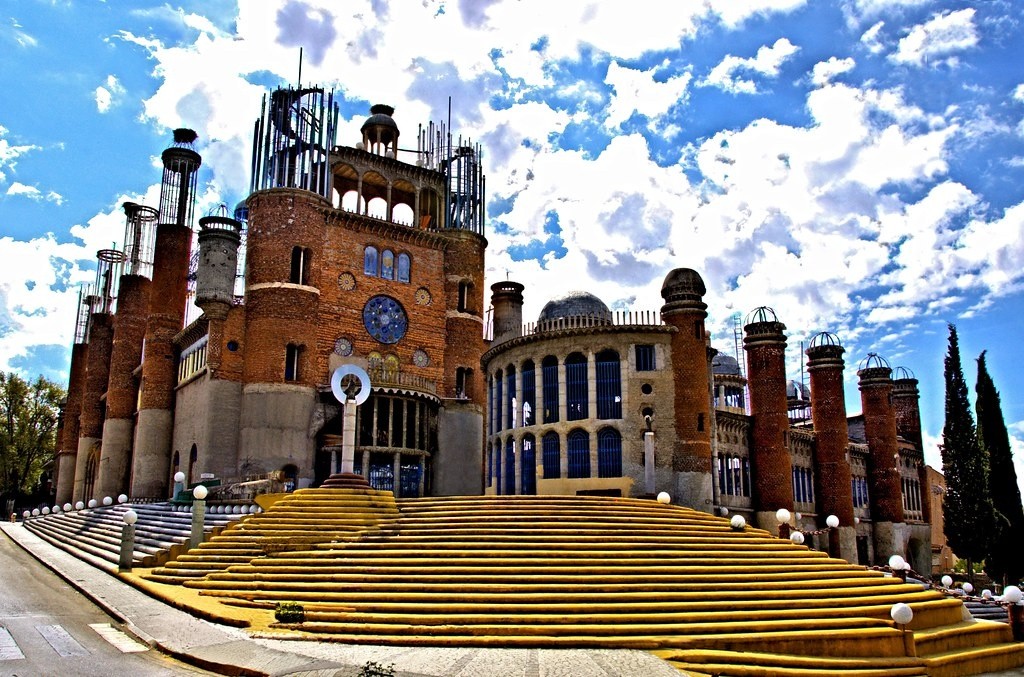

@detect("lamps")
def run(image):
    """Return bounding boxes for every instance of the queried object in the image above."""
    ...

[891,603,913,624]
[731,515,745,527]
[796,513,802,520]
[889,555,904,570]
[962,582,973,593]
[23,494,127,517]
[174,471,186,483]
[982,589,991,599]
[1004,586,1022,603]
[123,510,137,525]
[193,484,208,500]
[942,575,953,586]
[854,517,860,524]
[356,142,424,166]
[904,561,911,573]
[776,508,791,523]
[826,515,840,527]
[657,492,671,504]
[790,531,804,545]
[172,505,259,514]
